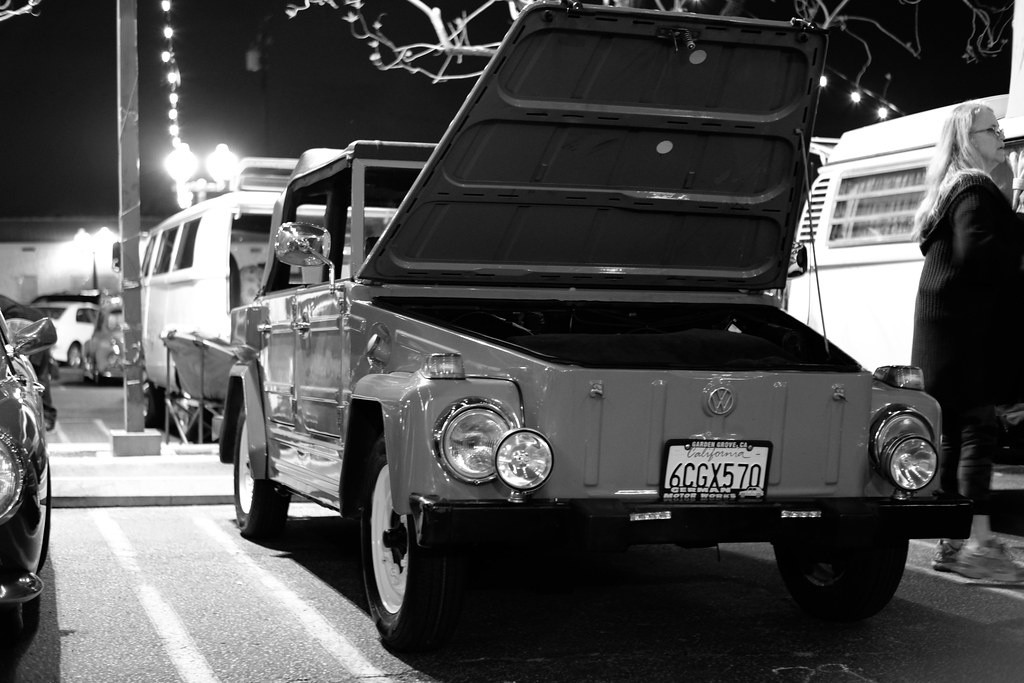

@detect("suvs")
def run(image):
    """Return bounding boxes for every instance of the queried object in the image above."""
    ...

[221,0,973,651]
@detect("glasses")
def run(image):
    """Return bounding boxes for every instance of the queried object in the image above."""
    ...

[969,122,1004,139]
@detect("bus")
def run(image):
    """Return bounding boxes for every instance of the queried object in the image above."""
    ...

[112,155,299,444]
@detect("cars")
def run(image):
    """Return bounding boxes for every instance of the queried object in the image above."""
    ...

[0,287,127,627]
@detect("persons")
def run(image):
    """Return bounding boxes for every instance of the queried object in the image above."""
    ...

[0,293,58,432]
[910,102,1024,584]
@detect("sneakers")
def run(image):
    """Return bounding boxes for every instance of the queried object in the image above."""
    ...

[931,537,1024,587]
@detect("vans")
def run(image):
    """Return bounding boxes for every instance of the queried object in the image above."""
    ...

[781,88,1023,373]
